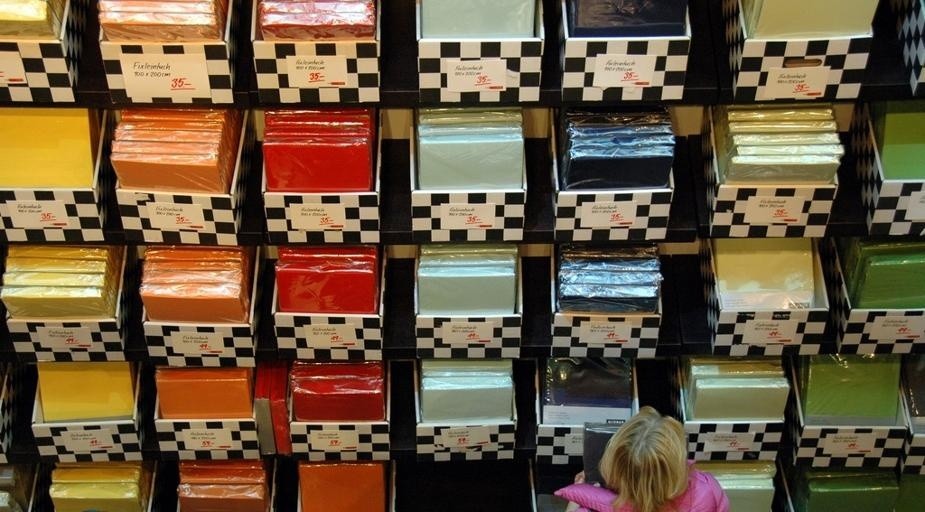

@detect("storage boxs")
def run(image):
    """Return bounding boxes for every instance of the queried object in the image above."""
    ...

[671,0,878,511]
[532,0,690,512]
[251,0,396,512]
[0,0,159,511]
[413,0,544,460]
[97,0,276,512]
[780,0,925,512]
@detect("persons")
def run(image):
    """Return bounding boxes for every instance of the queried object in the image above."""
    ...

[554,406,728,511]
